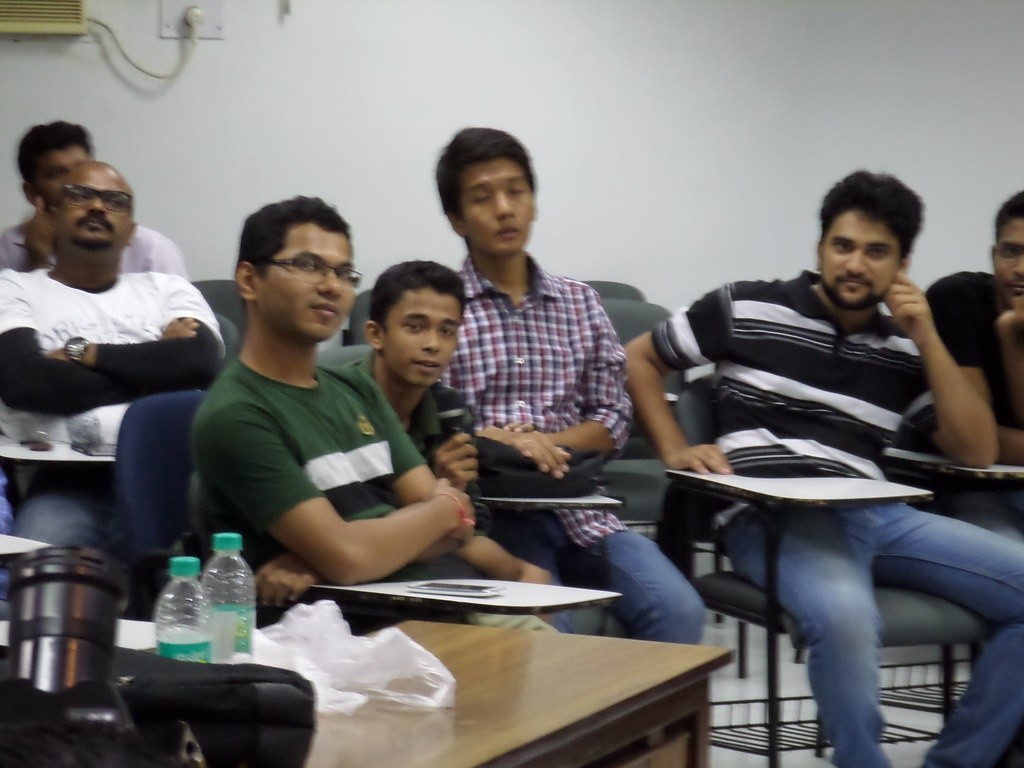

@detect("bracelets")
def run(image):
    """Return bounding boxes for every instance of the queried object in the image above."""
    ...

[440,492,468,535]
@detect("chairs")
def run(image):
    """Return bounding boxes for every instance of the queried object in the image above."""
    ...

[116,389,210,591]
[191,279,244,361]
[676,389,986,768]
[601,297,725,625]
[582,279,645,303]
[316,288,375,365]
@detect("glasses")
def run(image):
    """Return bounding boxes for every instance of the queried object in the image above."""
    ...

[61,185,133,213]
[264,253,362,288]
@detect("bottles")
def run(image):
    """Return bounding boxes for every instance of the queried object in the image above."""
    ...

[154,557,213,665]
[200,533,257,666]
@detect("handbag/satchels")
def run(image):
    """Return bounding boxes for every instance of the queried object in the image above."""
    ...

[475,432,614,499]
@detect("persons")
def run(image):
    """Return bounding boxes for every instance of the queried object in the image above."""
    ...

[432,128,706,646]
[350,259,558,635]
[0,120,226,602]
[619,171,1024,768]
[189,196,475,639]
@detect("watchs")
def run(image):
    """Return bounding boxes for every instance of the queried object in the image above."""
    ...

[65,337,89,364]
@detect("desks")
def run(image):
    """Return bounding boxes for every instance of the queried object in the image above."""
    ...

[665,467,938,510]
[0,430,116,471]
[309,578,623,615]
[943,460,1024,483]
[478,493,623,511]
[180,619,736,768]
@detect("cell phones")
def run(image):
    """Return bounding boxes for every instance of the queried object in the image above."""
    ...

[408,582,508,597]
